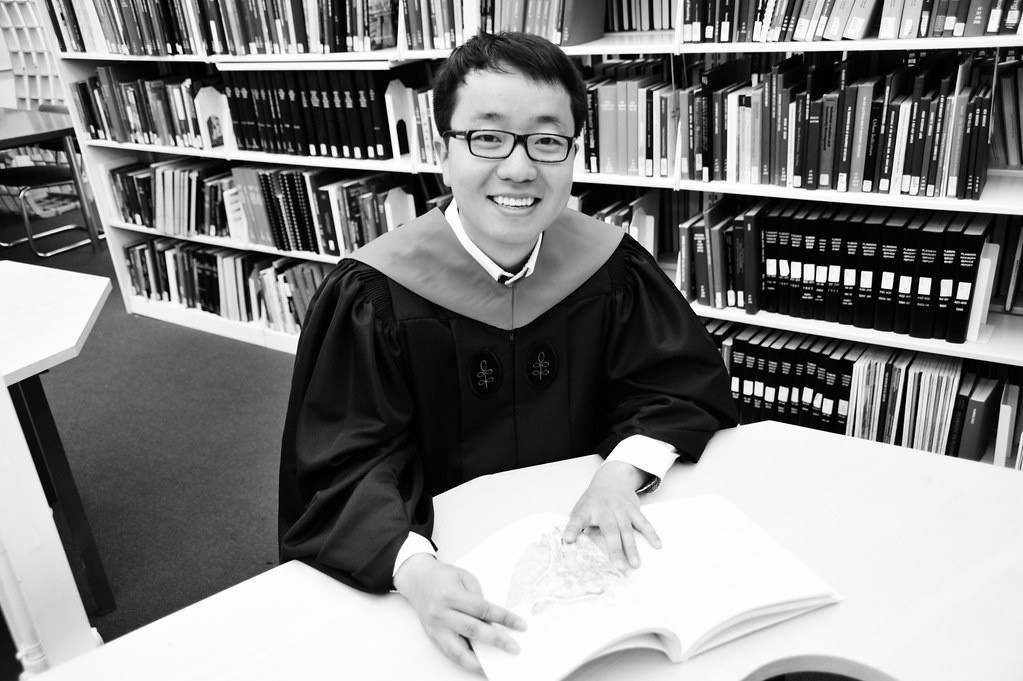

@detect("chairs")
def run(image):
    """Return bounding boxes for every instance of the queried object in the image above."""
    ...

[0,104,106,258]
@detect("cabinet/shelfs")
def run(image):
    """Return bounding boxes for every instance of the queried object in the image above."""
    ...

[0,0,93,202]
[40,0,1023,366]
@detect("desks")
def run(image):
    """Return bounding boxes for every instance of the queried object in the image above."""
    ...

[20,420,1022,681]
[0,107,102,255]
[2,260,117,617]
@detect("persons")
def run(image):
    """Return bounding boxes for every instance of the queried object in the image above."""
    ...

[279,32,739,676]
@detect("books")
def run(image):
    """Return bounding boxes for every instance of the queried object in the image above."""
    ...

[431,455,840,681]
[43,0,1023,472]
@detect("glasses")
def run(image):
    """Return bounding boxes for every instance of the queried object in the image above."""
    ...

[441,128,576,162]
